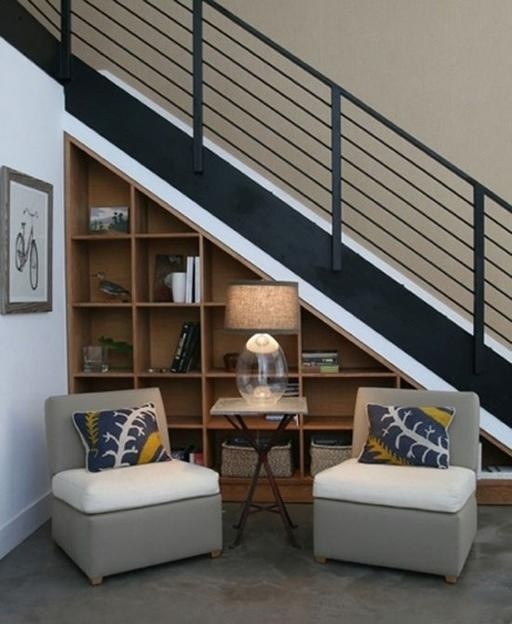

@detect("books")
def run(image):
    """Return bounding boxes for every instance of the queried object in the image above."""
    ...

[184,442,203,464]
[261,378,297,423]
[302,349,342,375]
[169,318,201,374]
[193,254,201,303]
[152,252,183,302]
[185,254,195,303]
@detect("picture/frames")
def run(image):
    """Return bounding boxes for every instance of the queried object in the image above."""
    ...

[1,164,52,316]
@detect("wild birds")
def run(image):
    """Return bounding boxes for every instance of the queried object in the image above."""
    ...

[92,271,131,303]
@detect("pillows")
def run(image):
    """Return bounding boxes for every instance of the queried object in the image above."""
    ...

[358,402,455,469]
[71,401,171,472]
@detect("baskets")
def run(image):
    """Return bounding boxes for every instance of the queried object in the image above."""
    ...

[220,440,294,479]
[309,435,353,478]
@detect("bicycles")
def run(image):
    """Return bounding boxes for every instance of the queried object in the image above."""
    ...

[15,208,41,289]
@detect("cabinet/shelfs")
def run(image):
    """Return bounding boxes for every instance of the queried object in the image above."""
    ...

[58,130,512,504]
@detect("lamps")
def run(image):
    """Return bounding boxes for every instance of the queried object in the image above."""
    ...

[225,280,302,409]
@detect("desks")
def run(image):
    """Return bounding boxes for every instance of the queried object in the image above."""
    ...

[210,397,309,551]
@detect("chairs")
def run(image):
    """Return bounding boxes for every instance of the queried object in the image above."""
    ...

[42,387,225,585]
[313,387,482,583]
